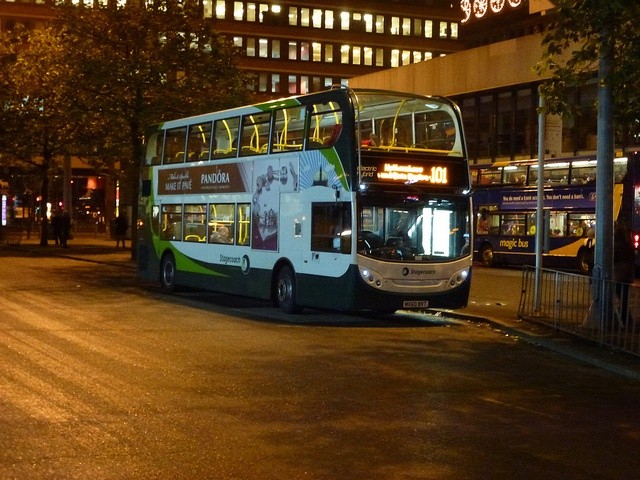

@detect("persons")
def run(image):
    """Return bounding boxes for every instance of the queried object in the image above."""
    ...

[580,173,589,184]
[553,228,560,236]
[576,221,587,237]
[520,175,526,184]
[356,131,380,148]
[210,227,231,244]
[477,208,490,233]
[252,165,274,221]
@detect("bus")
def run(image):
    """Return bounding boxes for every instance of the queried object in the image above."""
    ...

[136,83,474,313]
[468,150,640,276]
